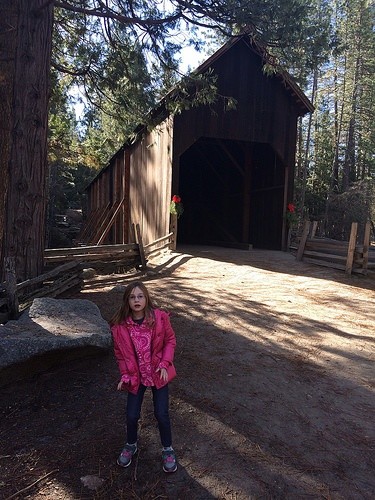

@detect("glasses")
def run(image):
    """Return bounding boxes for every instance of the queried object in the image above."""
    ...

[128,294,146,300]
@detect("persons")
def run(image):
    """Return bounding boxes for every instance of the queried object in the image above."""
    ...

[110,281,177,473]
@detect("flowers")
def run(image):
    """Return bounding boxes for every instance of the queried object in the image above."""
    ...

[170,195,181,218]
[288,203,297,222]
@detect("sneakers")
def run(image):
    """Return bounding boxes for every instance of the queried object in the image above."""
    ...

[162,447,178,473]
[117,442,138,467]
